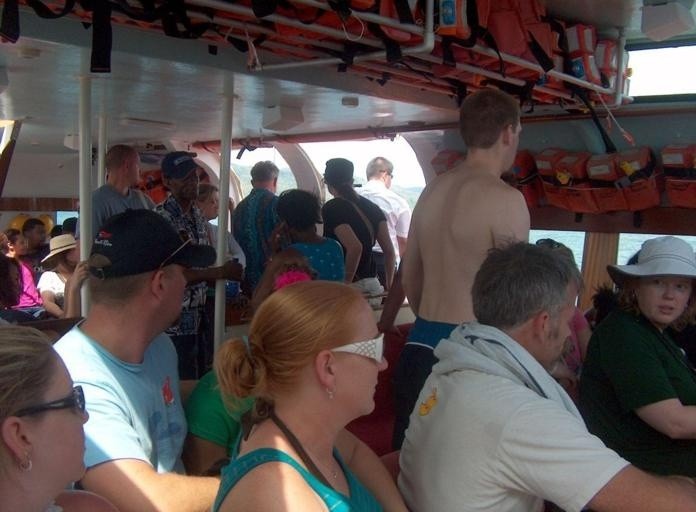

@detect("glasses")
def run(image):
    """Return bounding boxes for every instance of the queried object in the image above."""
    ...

[159,228,195,270]
[329,331,387,367]
[13,384,86,419]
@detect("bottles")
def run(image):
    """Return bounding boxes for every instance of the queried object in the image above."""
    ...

[226,256,240,297]
[196,312,213,371]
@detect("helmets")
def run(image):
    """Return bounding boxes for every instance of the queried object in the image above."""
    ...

[605,235,696,297]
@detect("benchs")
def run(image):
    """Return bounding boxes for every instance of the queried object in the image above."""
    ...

[347,320,418,457]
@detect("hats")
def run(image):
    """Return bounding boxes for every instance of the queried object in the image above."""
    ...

[161,150,203,182]
[40,233,78,263]
[87,206,217,281]
[323,157,354,184]
